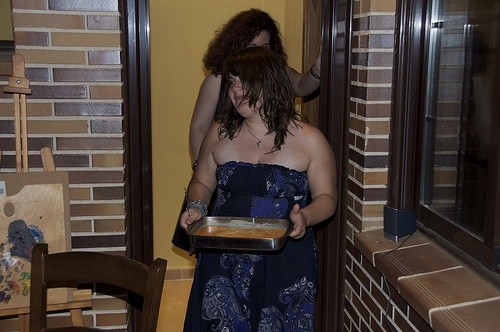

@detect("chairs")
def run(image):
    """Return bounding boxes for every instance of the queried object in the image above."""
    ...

[29,242,167,332]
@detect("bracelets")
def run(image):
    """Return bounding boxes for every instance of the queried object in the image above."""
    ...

[186,200,208,218]
[190,160,199,170]
[310,64,321,80]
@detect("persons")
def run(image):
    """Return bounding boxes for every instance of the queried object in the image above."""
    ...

[179,46,337,332]
[244,122,272,149]
[189,9,321,188]
